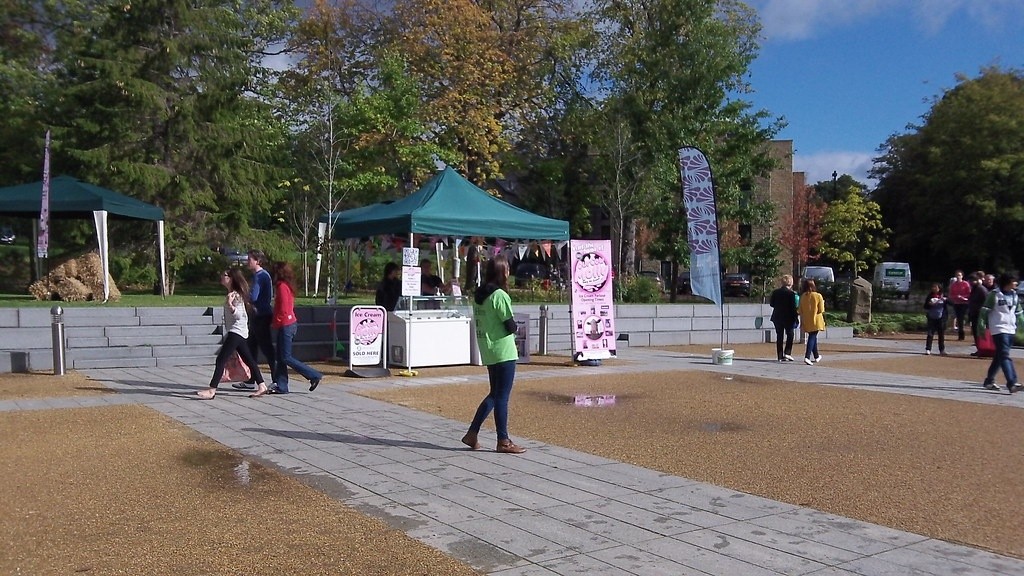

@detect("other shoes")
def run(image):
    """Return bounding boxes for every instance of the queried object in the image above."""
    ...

[266,388,282,394]
[815,354,822,363]
[462,430,481,449]
[986,384,1001,390]
[497,439,528,453]
[194,390,215,399]
[249,389,269,398]
[779,358,786,362]
[926,349,931,355]
[804,358,814,366]
[970,351,981,356]
[1009,383,1024,394]
[309,377,320,391]
[784,355,794,361]
[939,351,951,356]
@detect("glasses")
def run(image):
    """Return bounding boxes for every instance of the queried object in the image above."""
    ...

[221,273,229,277]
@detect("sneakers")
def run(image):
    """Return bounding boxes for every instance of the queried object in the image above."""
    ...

[264,382,277,393]
[231,381,255,389]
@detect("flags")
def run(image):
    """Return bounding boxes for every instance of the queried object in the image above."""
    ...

[37,131,50,258]
[678,145,722,312]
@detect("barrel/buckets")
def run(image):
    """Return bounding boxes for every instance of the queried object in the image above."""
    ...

[712,348,722,364]
[717,350,734,364]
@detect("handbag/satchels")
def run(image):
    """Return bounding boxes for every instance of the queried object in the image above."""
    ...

[218,349,252,382]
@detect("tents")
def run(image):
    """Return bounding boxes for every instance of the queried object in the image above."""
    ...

[320,166,576,358]
[0,173,167,303]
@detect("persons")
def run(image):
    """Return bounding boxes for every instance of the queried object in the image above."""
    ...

[465,246,480,293]
[419,258,454,310]
[265,260,322,394]
[231,249,277,390]
[797,279,825,365]
[770,274,799,363]
[967,271,997,358]
[947,269,972,342]
[976,272,1024,393]
[922,282,948,355]
[375,261,403,312]
[195,266,268,399]
[462,253,529,452]
